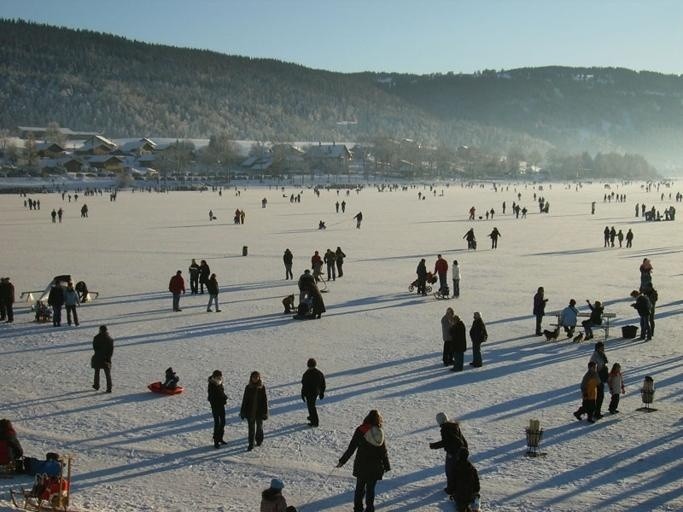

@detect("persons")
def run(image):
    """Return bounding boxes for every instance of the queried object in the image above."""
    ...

[1,175,683,512]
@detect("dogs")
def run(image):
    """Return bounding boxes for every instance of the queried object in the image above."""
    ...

[572,331,584,345]
[544,327,561,343]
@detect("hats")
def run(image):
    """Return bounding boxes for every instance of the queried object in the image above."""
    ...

[436,411,449,425]
[269,478,285,490]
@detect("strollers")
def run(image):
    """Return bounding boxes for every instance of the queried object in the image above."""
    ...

[407,273,439,295]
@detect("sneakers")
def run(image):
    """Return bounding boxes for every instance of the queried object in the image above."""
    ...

[534,330,656,342]
[572,408,620,425]
[442,360,483,373]
[90,383,113,395]
[212,438,263,450]
[1,318,80,326]
[305,415,319,427]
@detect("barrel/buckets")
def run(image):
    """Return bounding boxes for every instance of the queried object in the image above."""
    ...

[622,325,638,339]
